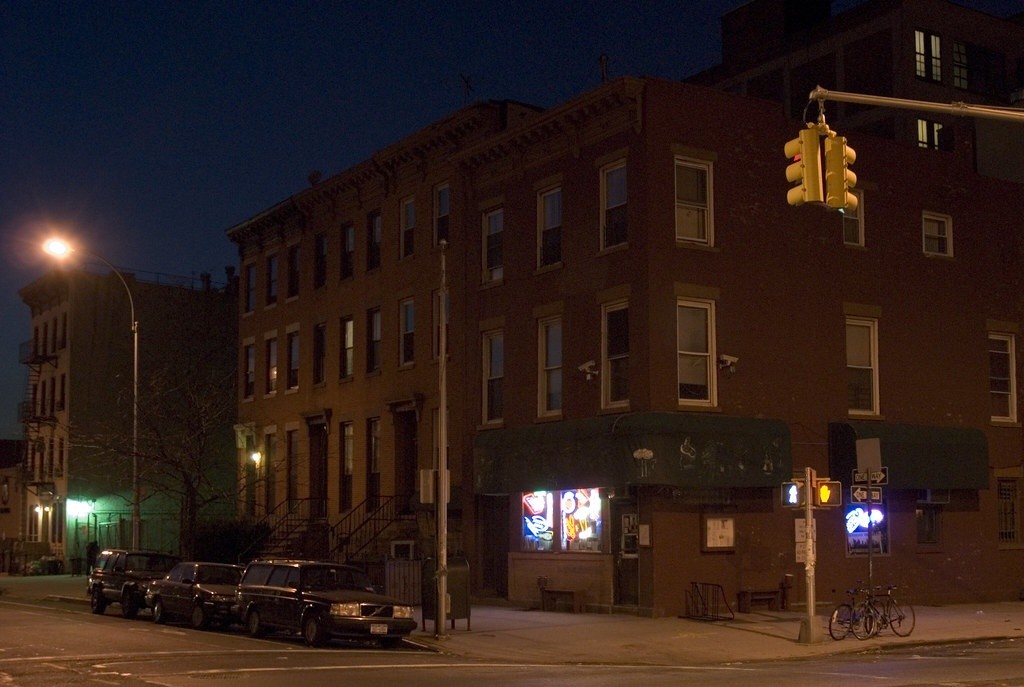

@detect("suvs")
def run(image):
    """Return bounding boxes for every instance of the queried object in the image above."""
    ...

[232,556,418,649]
[88,548,182,617]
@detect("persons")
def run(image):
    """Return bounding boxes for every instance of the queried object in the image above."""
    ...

[86,540,100,576]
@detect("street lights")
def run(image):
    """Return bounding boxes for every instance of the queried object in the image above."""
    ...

[44,240,139,546]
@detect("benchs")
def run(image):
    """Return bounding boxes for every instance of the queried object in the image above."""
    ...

[542,586,585,615]
[738,588,784,613]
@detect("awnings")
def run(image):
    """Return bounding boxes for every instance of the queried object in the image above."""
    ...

[830,420,989,490]
[475,413,792,493]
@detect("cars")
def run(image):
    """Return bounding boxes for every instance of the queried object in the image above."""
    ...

[144,561,246,629]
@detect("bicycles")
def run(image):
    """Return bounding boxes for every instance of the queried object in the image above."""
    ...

[831,581,916,640]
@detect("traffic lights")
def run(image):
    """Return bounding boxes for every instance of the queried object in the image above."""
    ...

[818,482,842,506]
[784,130,824,204]
[784,485,802,506]
[825,136,858,210]
